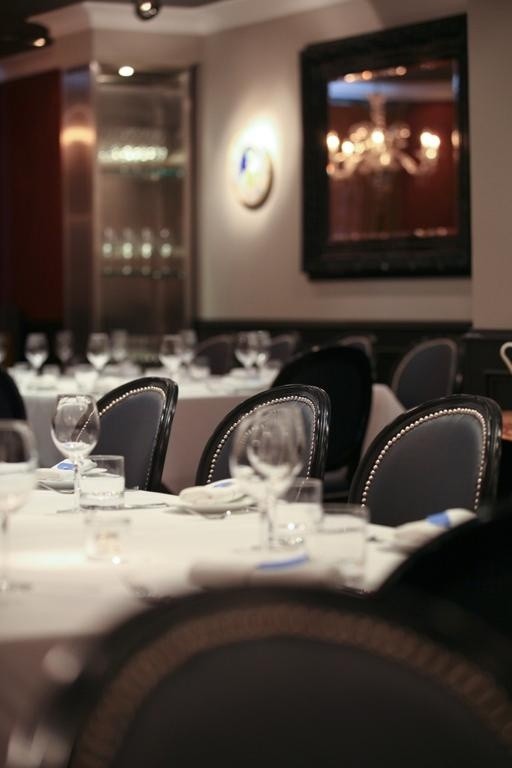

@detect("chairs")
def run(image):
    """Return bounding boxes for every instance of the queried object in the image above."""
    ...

[347,393,504,515]
[57,374,178,488]
[389,336,457,405]
[270,340,374,490]
[73,576,501,767]
[195,383,333,496]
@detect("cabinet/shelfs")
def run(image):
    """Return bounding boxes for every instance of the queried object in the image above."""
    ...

[57,83,195,321]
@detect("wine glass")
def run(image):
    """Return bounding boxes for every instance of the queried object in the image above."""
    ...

[97,123,174,280]
[1,393,312,592]
[0,330,273,386]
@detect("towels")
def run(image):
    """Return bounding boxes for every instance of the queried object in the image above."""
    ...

[177,486,246,506]
[393,508,477,553]
[35,458,97,481]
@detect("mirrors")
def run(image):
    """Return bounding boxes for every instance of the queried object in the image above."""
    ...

[299,13,475,278]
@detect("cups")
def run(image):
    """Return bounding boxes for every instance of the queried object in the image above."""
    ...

[77,455,372,591]
